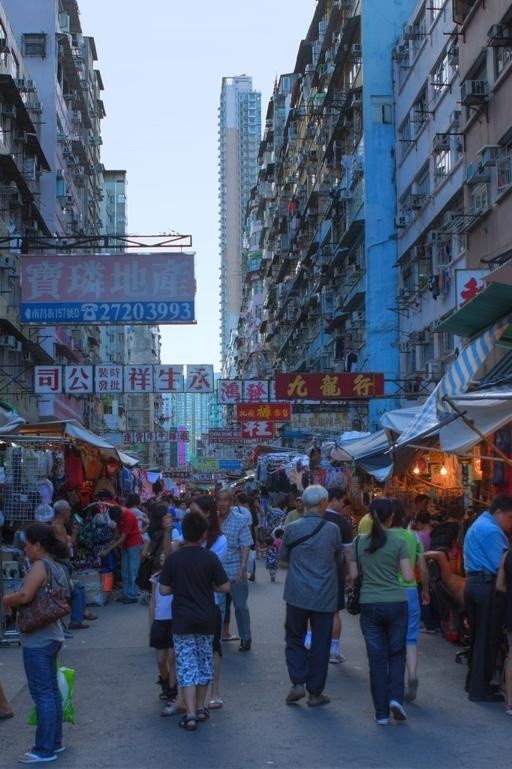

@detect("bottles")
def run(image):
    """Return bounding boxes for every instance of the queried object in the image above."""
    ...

[298,447,348,490]
[35,505,55,523]
[37,479,54,504]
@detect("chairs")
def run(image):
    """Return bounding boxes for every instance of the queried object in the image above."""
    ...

[83,611,97,620]
[286,652,417,726]
[469,694,504,702]
[69,622,89,628]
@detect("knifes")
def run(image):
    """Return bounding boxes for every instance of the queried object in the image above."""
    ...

[423,360,441,383]
[2,29,126,368]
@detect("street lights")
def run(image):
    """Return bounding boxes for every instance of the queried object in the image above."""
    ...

[27,667,77,725]
[135,556,152,589]
[18,588,71,632]
[347,576,361,615]
[69,459,120,551]
[147,503,168,543]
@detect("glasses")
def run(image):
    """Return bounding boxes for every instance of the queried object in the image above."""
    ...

[439,449,450,476]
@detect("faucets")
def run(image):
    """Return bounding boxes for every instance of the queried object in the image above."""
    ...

[157,635,252,731]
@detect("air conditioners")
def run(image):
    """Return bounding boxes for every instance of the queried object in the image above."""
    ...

[431,111,462,152]
[461,79,489,105]
[2,29,126,368]
[423,360,441,383]
[481,147,495,167]
[393,193,464,301]
[487,25,504,41]
[447,46,459,66]
[465,163,480,179]
[389,22,417,63]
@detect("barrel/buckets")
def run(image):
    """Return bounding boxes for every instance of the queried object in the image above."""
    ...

[18,745,65,762]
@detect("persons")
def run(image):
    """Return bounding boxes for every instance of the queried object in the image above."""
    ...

[163,495,232,715]
[1,687,16,720]
[214,490,253,650]
[277,483,343,706]
[1,523,73,763]
[387,499,424,700]
[141,503,185,699]
[350,497,417,727]
[408,494,478,643]
[159,511,233,732]
[320,485,352,663]
[48,482,306,640]
[464,494,511,716]
